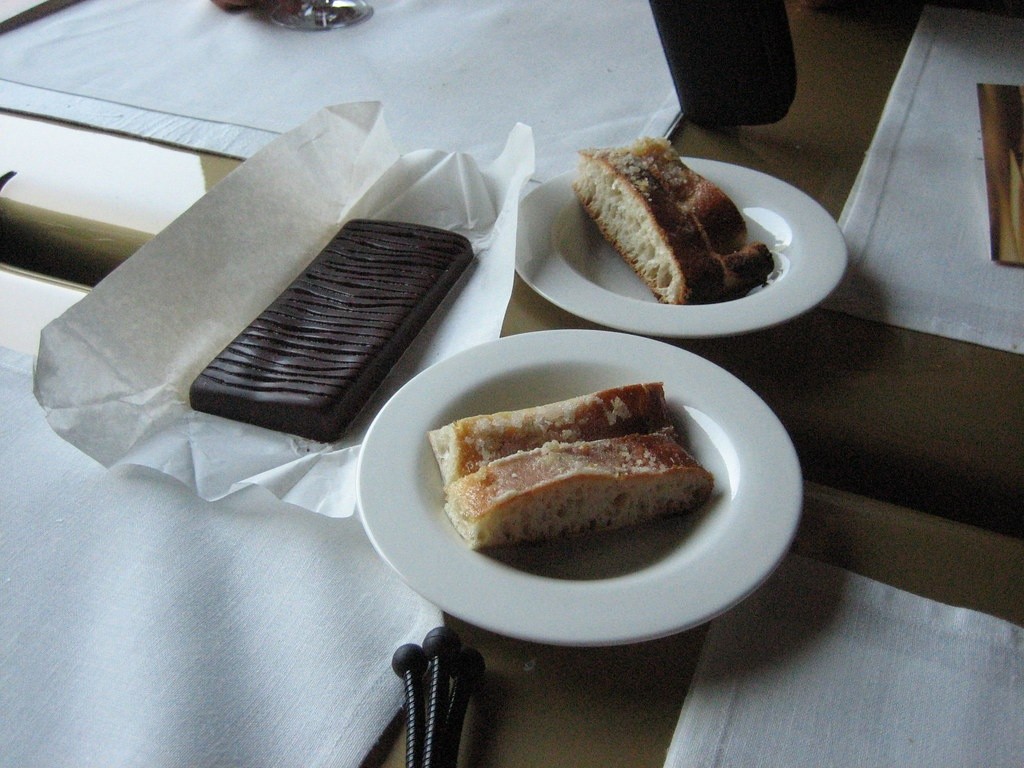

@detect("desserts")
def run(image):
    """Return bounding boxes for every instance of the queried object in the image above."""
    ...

[186,218,475,442]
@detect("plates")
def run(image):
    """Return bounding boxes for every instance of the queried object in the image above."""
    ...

[514,157,850,339]
[356,328,804,647]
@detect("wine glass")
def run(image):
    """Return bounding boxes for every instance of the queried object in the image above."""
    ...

[266,0,371,32]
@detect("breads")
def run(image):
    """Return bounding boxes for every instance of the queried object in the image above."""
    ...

[443,432,714,551]
[569,145,729,305]
[620,136,775,298]
[425,381,673,489]
